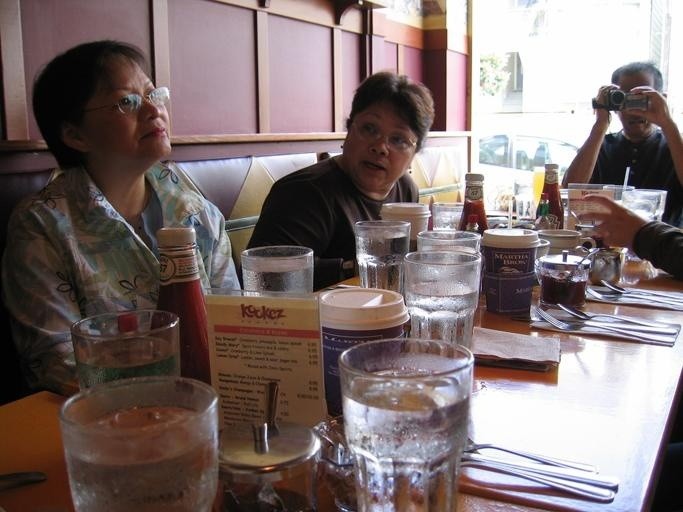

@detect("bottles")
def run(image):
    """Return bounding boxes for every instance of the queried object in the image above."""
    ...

[457,169,488,235]
[218,420,319,511]
[532,164,564,229]
[146,225,210,380]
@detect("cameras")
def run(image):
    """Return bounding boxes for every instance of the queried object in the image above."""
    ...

[592,89,648,111]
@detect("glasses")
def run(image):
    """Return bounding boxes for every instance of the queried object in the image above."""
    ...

[56,85,171,127]
[350,114,418,153]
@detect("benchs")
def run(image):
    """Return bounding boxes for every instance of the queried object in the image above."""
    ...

[2,128,479,407]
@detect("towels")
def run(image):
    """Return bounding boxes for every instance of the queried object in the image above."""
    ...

[463,320,563,374]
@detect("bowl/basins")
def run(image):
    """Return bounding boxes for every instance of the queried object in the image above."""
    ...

[311,414,360,511]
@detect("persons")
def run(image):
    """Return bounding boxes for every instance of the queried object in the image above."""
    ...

[561,63,683,228]
[238,70,436,291]
[577,193,683,281]
[0,40,243,395]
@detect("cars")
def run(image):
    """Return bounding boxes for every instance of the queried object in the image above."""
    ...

[479,135,582,186]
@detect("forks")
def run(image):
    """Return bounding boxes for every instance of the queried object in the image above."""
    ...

[531,279,682,348]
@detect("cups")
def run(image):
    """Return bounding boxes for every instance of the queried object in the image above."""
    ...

[70,308,180,388]
[239,244,315,298]
[604,184,668,263]
[315,286,410,421]
[56,375,220,511]
[352,202,658,361]
[337,335,475,511]
